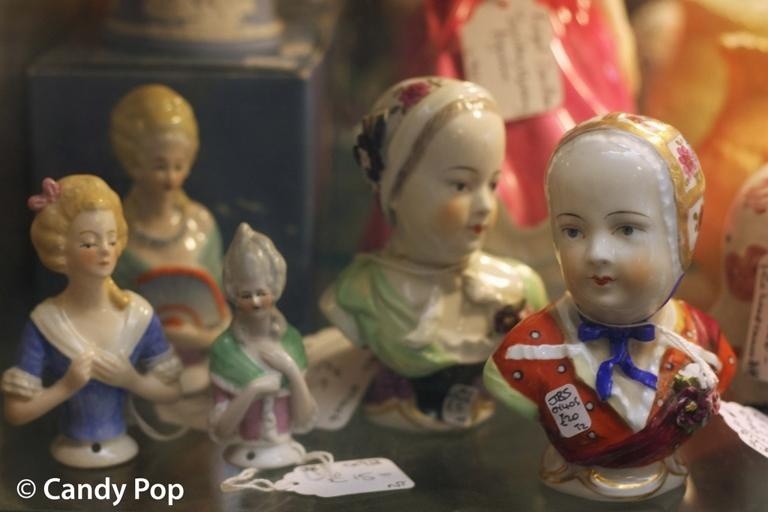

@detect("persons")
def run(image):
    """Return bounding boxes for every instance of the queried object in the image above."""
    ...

[481,111,741,501]
[107,79,225,433]
[1,172,186,469]
[202,224,315,468]
[311,72,552,435]
